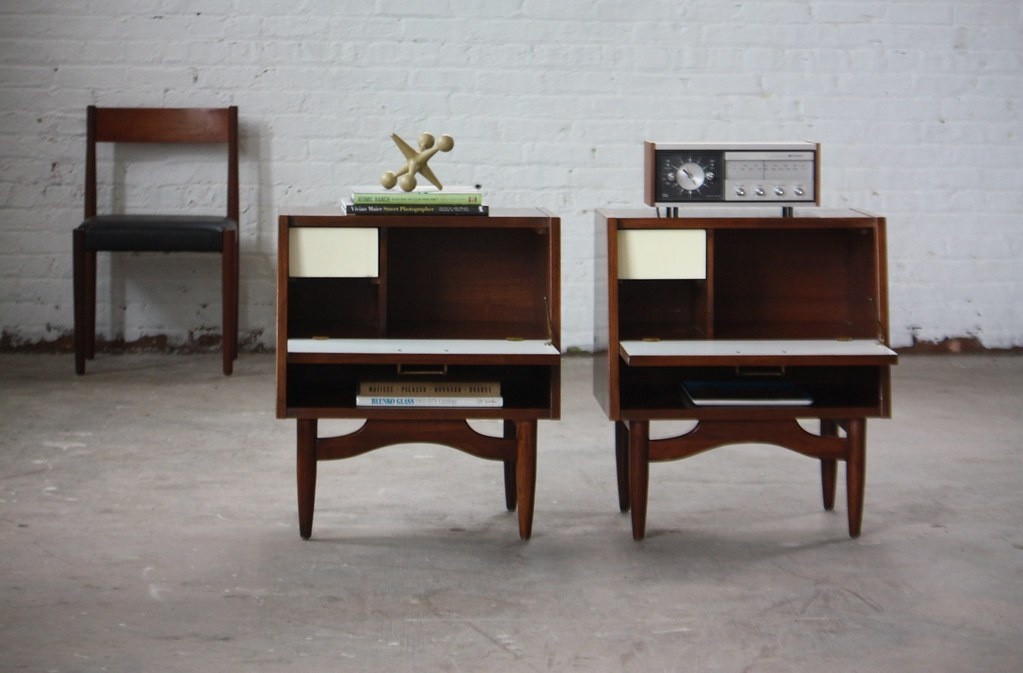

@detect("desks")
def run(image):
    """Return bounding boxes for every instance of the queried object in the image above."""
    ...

[274,212,561,541]
[592,202,898,539]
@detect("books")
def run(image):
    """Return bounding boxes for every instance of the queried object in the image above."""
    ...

[340,199,489,216]
[350,185,482,205]
[355,379,504,407]
[679,378,813,405]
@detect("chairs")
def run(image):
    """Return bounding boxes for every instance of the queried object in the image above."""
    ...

[71,105,241,378]
